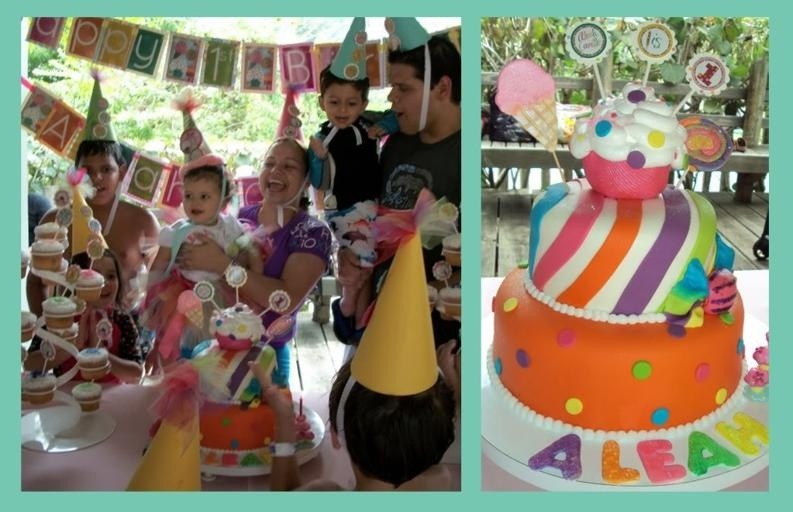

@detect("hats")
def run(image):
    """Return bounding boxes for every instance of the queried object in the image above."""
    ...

[178,111,223,180]
[125,392,202,490]
[350,228,456,398]
[329,16,369,81]
[71,184,109,257]
[84,78,116,142]
[272,90,308,150]
[385,16,433,53]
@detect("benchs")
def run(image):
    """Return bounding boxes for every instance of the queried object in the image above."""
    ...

[481,101,769,205]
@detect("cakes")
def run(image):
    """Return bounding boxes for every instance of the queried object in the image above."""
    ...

[41,264,80,329]
[34,190,70,243]
[21,311,37,343]
[72,382,102,412]
[481,20,769,487]
[21,346,27,402]
[427,203,461,321]
[77,318,112,379]
[175,265,315,468]
[75,239,107,303]
[30,207,74,268]
[24,341,58,405]
